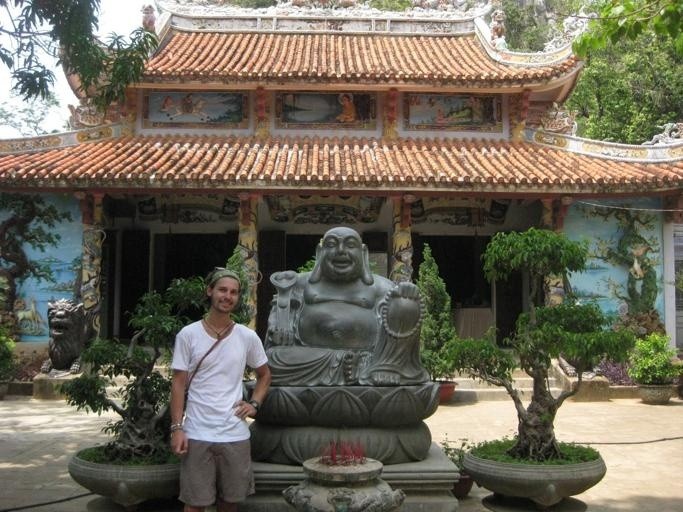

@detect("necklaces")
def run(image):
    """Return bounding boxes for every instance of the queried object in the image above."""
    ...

[203,317,232,339]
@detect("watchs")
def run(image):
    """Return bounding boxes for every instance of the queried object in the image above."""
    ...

[248,399,260,412]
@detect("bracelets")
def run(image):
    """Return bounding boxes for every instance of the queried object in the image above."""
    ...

[169,423,182,431]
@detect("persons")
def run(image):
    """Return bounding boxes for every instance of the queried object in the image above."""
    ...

[334,95,356,123]
[256,225,432,385]
[182,93,192,107]
[160,95,173,113]
[168,266,271,510]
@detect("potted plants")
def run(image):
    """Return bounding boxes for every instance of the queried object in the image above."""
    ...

[423,228,635,509]
[67,275,219,512]
[0,315,19,400]
[563,195,668,329]
[627,331,682,404]
[420,246,463,402]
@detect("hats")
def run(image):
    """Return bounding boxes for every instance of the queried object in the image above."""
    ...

[207,270,243,287]
